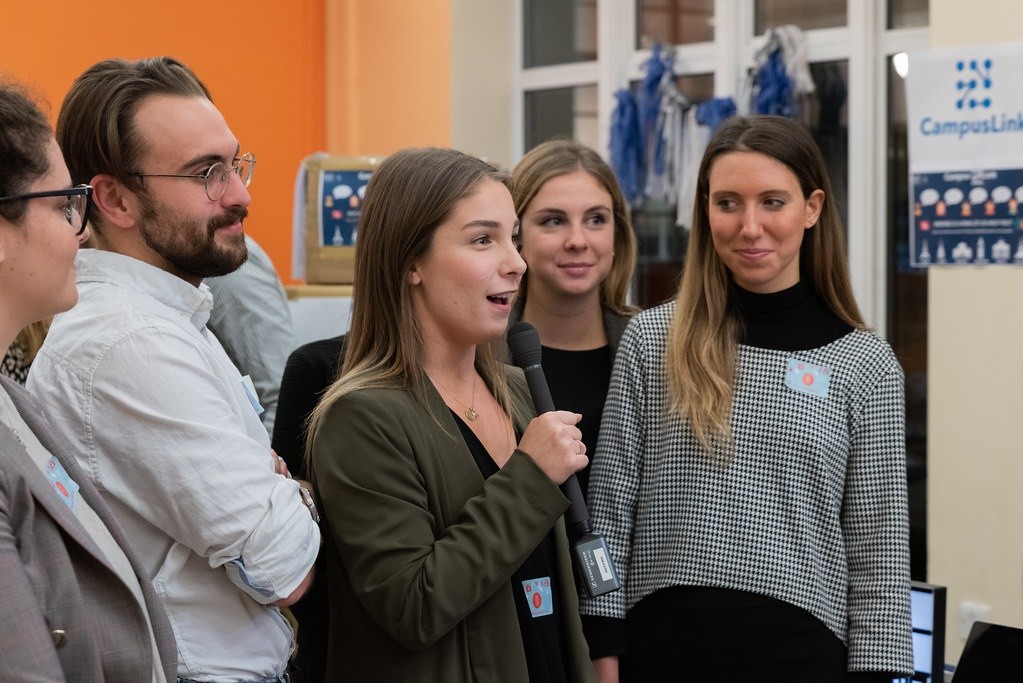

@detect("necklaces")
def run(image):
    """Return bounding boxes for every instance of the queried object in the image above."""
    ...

[420,365,479,422]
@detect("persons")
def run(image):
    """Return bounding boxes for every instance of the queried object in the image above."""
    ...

[302,148,608,682]
[1,54,322,682]
[271,324,348,485]
[577,117,916,683]
[497,138,644,498]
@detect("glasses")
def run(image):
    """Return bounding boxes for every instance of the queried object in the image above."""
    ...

[1,183,94,235]
[113,153,257,201]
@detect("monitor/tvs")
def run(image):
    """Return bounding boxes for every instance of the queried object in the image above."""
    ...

[892,579,949,683]
[306,154,385,287]
[946,618,1023,683]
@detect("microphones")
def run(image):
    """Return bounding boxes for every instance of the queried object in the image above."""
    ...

[507,322,592,536]
